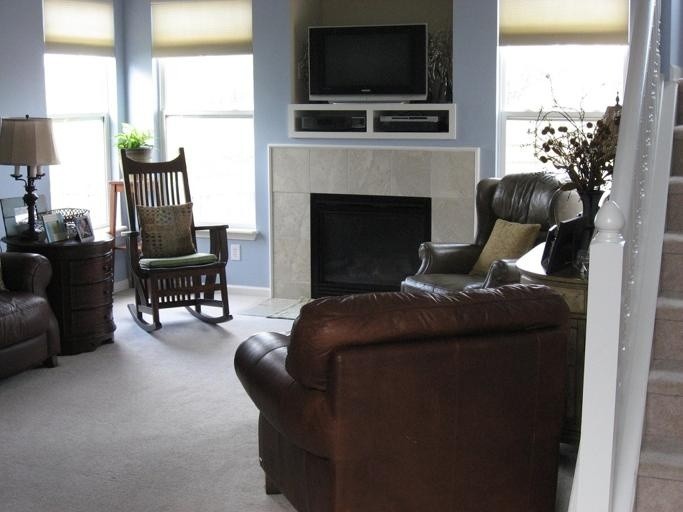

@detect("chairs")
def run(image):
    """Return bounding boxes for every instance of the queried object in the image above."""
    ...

[120,147,232,334]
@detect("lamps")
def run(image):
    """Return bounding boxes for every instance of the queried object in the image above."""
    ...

[600,91,622,168]
[0,113,60,240]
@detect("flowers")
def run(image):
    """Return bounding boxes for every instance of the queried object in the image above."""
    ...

[520,75,620,203]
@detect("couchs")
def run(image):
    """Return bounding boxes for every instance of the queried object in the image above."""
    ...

[233,283,571,512]
[400,171,583,293]
[0,252,60,380]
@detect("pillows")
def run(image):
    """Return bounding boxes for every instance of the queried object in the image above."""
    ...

[467,218,540,276]
[136,202,196,259]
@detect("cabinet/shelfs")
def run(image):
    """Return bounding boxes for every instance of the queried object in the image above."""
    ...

[2,230,116,357]
[105,178,159,299]
[514,242,588,449]
[285,103,456,139]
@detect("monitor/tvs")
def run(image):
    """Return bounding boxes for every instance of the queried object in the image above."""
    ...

[306,25,429,105]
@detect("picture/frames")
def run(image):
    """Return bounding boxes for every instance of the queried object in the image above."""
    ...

[545,215,583,275]
[41,213,68,243]
[0,194,47,238]
[73,215,94,243]
[540,225,558,265]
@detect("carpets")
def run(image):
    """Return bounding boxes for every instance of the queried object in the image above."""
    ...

[236,297,316,320]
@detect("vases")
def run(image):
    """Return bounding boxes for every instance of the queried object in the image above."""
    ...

[571,190,604,261]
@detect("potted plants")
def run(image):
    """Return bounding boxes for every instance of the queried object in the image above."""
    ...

[111,123,160,179]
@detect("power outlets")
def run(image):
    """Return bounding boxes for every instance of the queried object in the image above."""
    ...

[230,243,240,261]
